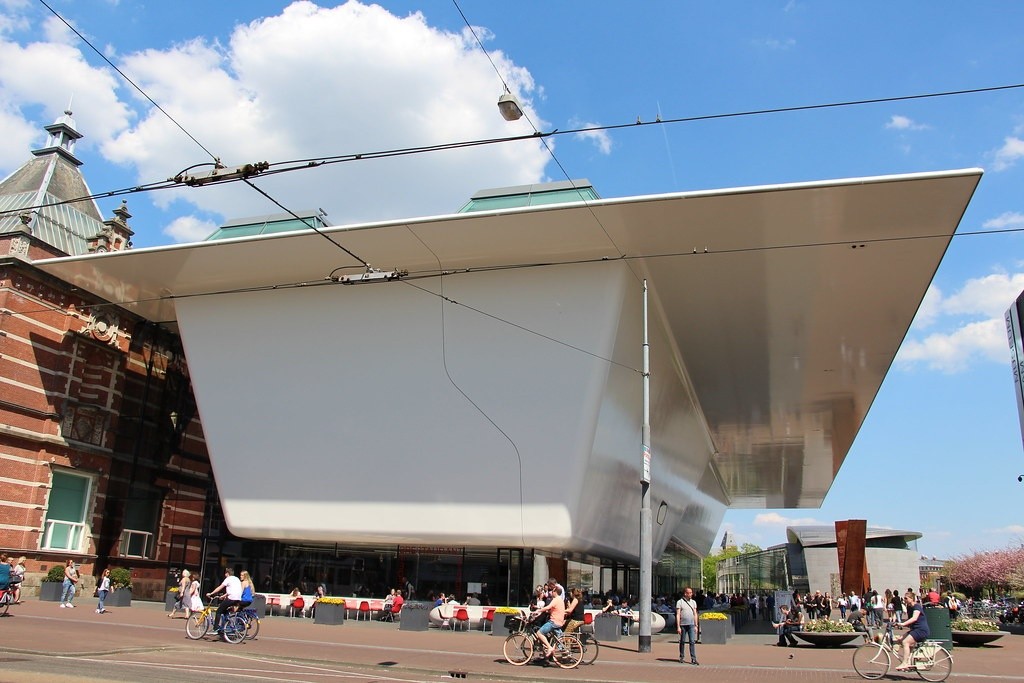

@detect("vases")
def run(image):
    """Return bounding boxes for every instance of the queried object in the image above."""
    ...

[795,630,861,646]
[951,631,1011,646]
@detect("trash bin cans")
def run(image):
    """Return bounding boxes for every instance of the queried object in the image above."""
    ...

[922,602,953,650]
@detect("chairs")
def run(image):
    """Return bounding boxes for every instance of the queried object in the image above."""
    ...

[584,612,593,633]
[266,595,496,632]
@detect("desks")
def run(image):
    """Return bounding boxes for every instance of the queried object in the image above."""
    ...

[617,614,636,638]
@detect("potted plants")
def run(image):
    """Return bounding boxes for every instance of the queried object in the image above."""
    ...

[314,597,345,625]
[253,594,266,618]
[104,566,134,607]
[699,605,751,643]
[39,565,68,601]
[400,601,428,630]
[165,587,179,611]
[493,607,521,636]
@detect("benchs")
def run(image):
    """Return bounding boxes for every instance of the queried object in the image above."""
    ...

[584,609,666,635]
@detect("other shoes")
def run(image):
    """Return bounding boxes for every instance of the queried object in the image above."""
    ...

[788,640,798,647]
[66,602,74,608]
[772,641,788,647]
[679,658,685,663]
[691,658,699,666]
[895,664,909,670]
[167,614,173,619]
[184,616,188,619]
[95,607,105,614]
[60,603,66,608]
[546,646,554,657]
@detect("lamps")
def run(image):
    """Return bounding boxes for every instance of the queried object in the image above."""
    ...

[497,88,525,121]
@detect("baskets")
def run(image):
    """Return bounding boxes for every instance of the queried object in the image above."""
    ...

[505,615,525,631]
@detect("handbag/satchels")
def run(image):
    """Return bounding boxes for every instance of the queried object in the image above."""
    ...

[174,592,183,601]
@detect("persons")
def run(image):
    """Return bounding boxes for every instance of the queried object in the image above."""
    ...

[926,588,961,621]
[290,587,300,599]
[797,588,913,630]
[652,592,683,613]
[0,551,27,603]
[207,568,255,635]
[583,590,639,615]
[773,605,804,647]
[526,578,585,662]
[403,577,415,600]
[167,569,204,619]
[896,592,930,671]
[379,589,403,621]
[676,588,699,666]
[60,559,78,608]
[695,590,774,621]
[93,569,115,614]
[299,576,308,595]
[313,585,325,599]
[434,593,481,608]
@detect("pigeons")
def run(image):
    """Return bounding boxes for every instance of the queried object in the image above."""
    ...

[790,653,794,658]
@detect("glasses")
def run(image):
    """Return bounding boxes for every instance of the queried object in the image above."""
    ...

[904,597,909,600]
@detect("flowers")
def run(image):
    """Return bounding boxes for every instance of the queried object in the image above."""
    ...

[951,619,999,631]
[595,613,621,641]
[802,615,855,632]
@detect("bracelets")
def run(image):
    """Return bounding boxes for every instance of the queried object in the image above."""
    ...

[695,625,698,627]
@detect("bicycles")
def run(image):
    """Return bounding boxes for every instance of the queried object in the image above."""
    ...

[852,621,954,682]
[502,610,601,669]
[185,592,261,645]
